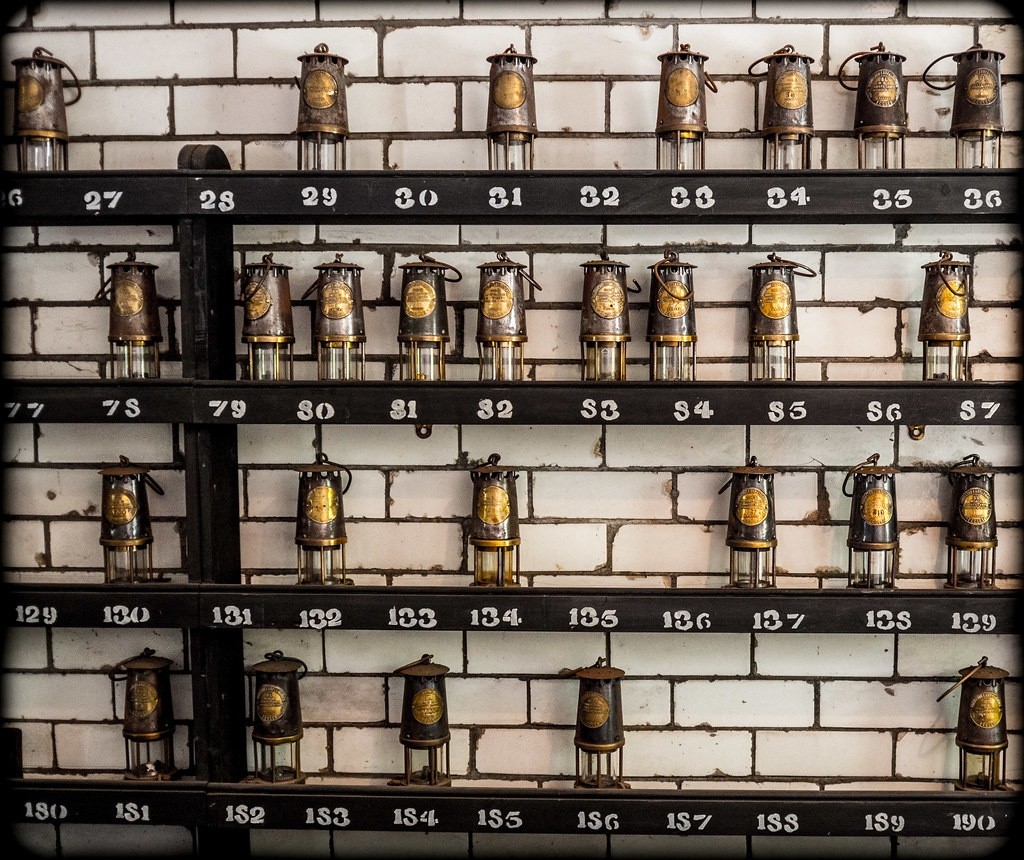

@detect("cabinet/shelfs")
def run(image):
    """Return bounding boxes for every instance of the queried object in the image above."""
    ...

[0,144,1024,859]
